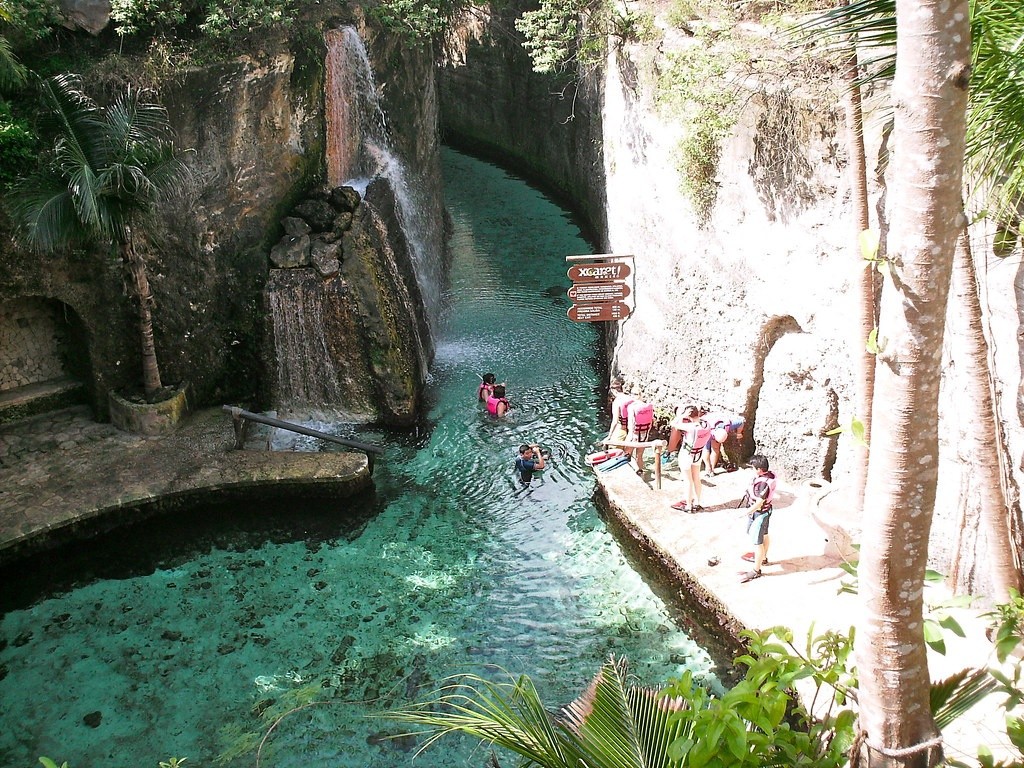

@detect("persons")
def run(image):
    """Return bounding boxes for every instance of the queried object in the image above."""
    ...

[517,444,545,484]
[477,370,509,417]
[738,455,778,585]
[602,378,745,514]
